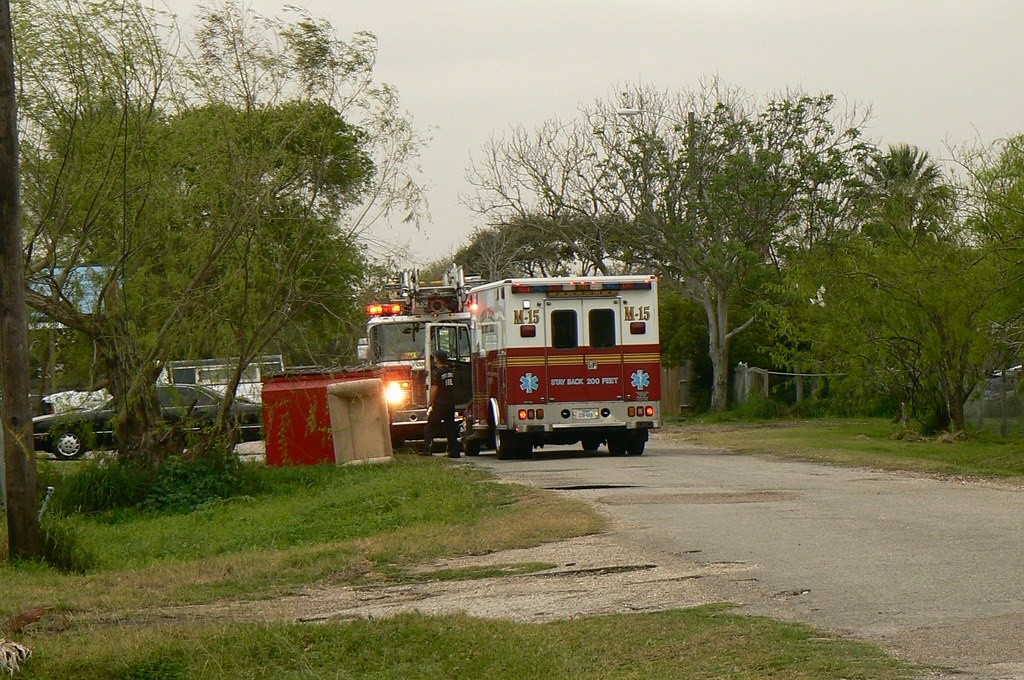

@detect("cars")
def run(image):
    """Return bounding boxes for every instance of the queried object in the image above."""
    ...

[32,383,265,461]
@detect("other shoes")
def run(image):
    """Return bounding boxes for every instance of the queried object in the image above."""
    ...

[444,452,460,457]
[418,450,430,455]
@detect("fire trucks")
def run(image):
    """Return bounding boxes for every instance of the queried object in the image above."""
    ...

[425,272,661,460]
[357,266,484,448]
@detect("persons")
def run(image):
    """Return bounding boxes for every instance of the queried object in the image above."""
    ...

[415,349,457,457]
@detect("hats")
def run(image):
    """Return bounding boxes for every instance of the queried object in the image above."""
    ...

[432,350,447,359]
